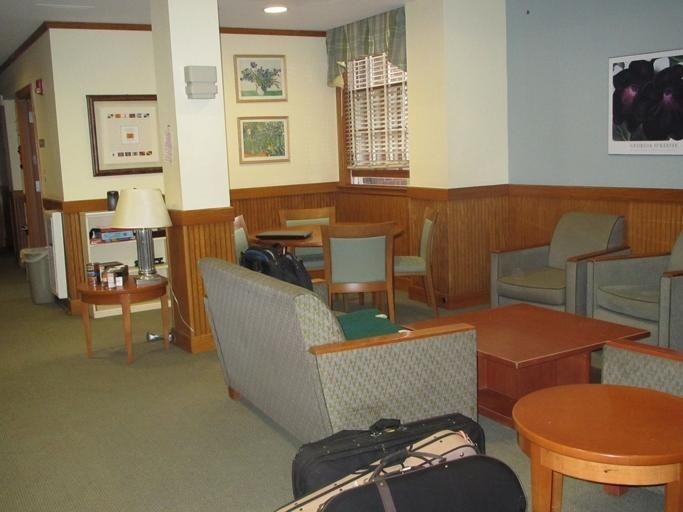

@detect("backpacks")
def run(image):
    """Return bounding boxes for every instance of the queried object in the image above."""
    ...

[242,242,313,292]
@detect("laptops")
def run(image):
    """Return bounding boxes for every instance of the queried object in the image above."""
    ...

[256,229,312,239]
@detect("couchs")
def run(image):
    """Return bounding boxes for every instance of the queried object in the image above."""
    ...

[195,254,480,450]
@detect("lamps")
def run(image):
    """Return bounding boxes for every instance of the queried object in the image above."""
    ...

[111,186,173,277]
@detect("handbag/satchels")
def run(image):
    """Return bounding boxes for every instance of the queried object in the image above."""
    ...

[275,430,480,512]
[317,450,526,512]
[291,413,486,512]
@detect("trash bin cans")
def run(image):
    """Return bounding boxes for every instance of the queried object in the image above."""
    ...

[22,246,56,305]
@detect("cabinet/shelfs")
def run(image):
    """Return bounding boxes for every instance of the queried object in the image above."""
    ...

[79,210,171,319]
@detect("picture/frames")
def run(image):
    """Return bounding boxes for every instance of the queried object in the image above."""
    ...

[234,53,289,103]
[607,48,683,156]
[237,115,290,165]
[85,94,165,178]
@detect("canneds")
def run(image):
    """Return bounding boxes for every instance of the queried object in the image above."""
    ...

[86,262,99,289]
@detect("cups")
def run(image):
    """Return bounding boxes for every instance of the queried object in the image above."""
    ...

[107,190,118,211]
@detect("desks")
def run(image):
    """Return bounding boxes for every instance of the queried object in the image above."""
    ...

[509,382,683,512]
[78,276,174,364]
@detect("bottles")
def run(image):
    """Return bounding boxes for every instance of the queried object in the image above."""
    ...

[101,267,125,287]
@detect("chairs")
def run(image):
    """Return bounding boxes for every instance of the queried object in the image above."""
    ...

[593,332,683,405]
[489,209,631,316]
[233,199,444,326]
[586,228,683,352]
